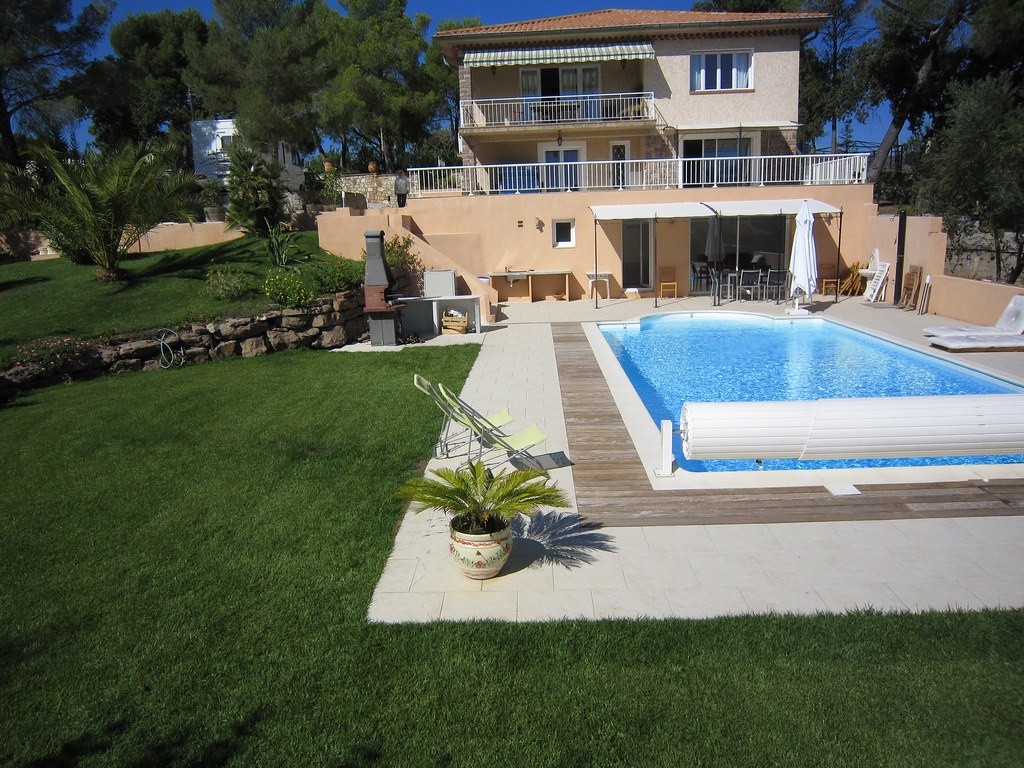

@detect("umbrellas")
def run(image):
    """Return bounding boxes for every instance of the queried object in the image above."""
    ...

[704,218,726,270]
[789,199,818,311]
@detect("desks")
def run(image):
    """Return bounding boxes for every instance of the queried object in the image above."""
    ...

[529,103,579,122]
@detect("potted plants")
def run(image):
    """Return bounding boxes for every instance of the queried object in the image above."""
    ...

[197,177,226,222]
[300,164,344,214]
[395,460,572,580]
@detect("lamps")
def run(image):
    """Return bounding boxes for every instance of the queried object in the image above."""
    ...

[558,130,562,147]
[536,217,540,229]
[621,60,627,69]
[491,66,497,75]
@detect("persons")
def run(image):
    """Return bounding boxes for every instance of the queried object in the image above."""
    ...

[395,170,410,208]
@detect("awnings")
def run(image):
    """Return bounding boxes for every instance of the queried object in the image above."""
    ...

[671,120,804,130]
[463,40,655,68]
[588,198,844,222]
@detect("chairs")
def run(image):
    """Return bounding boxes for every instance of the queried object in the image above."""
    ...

[897,266,924,312]
[818,265,840,296]
[412,372,515,460]
[922,295,1024,352]
[864,262,891,303]
[658,266,678,299]
[690,262,789,305]
[438,383,551,479]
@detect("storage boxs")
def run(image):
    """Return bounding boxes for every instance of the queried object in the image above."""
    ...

[443,310,469,335]
